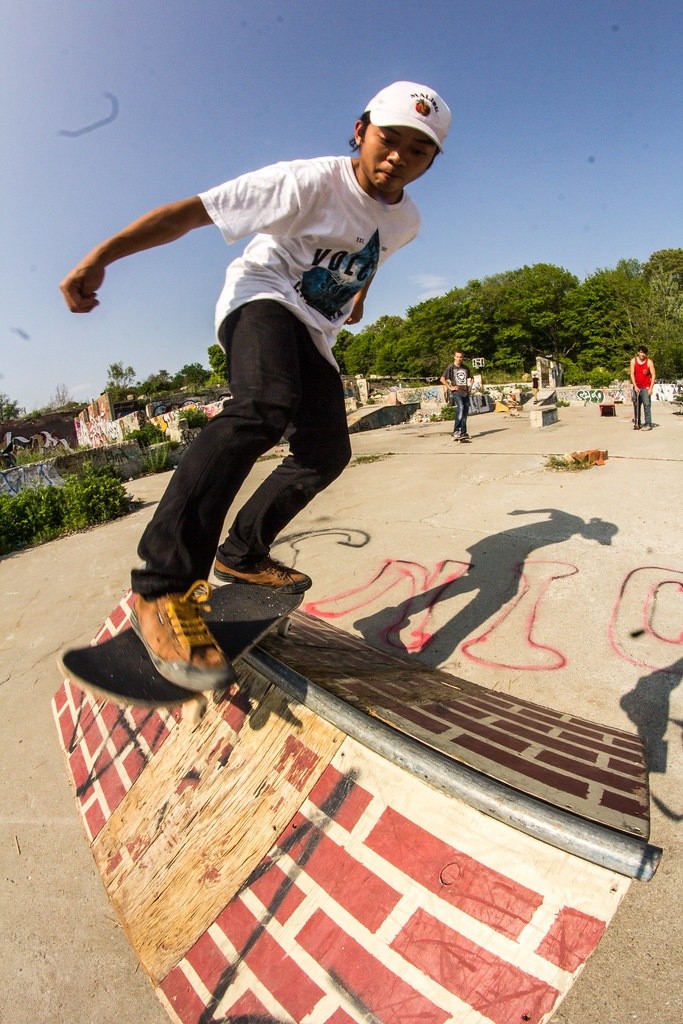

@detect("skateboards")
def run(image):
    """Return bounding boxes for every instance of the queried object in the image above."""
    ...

[62,582,306,707]
[634,392,641,430]
[451,435,473,443]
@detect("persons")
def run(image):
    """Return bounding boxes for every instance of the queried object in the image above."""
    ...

[532,374,540,404]
[440,350,474,439]
[59,80,453,690]
[630,345,655,431]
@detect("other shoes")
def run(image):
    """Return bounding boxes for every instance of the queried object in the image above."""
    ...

[633,422,641,430]
[451,430,468,439]
[641,423,652,430]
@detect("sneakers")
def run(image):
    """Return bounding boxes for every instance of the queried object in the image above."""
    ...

[214,555,313,593]
[130,580,236,691]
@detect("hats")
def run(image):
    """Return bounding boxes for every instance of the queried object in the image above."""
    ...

[364,80,451,154]
